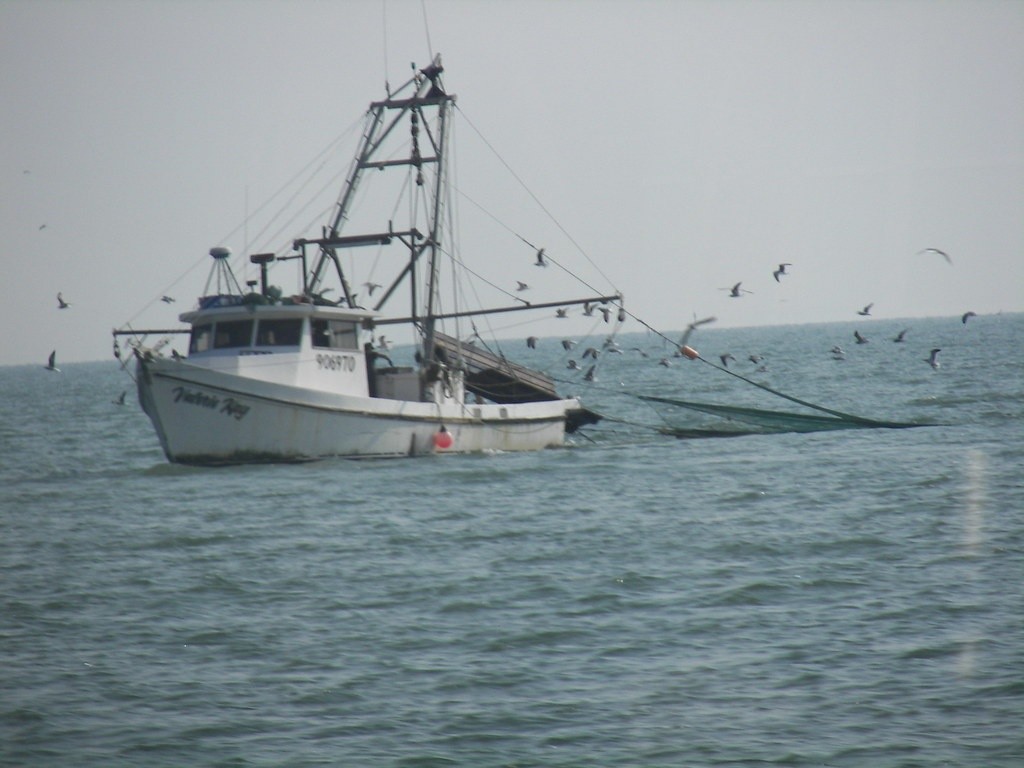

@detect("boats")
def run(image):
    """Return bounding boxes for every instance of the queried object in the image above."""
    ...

[110,51,625,465]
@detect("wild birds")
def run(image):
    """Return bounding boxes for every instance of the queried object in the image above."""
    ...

[41,247,977,408]
